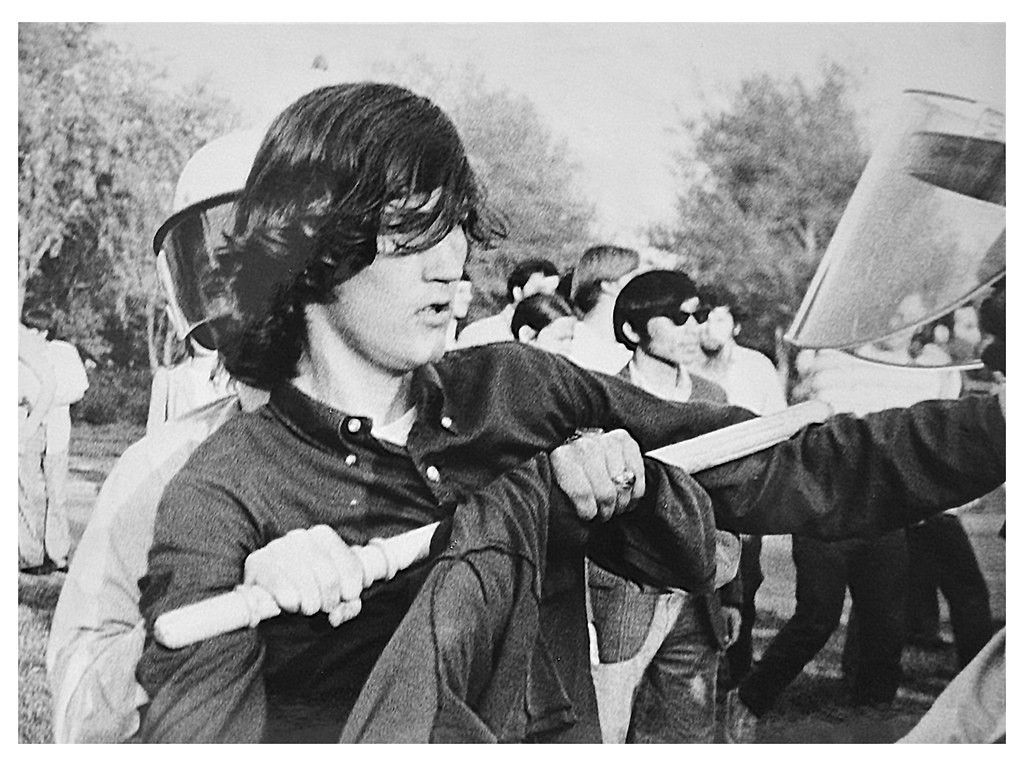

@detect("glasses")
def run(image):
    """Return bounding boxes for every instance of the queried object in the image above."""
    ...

[669,305,710,327]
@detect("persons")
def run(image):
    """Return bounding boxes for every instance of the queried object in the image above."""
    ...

[23,295,90,573]
[45,126,646,743]
[441,245,995,743]
[18,322,47,575]
[128,84,1006,744]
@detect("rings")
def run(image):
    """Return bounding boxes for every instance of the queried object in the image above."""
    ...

[613,468,635,491]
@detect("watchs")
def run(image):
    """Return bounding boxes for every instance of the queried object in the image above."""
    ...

[559,426,605,447]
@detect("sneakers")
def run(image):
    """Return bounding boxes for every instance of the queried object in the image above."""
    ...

[726,689,756,744]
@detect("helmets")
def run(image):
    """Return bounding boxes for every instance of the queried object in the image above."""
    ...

[790,36,1006,369]
[156,133,264,340]
